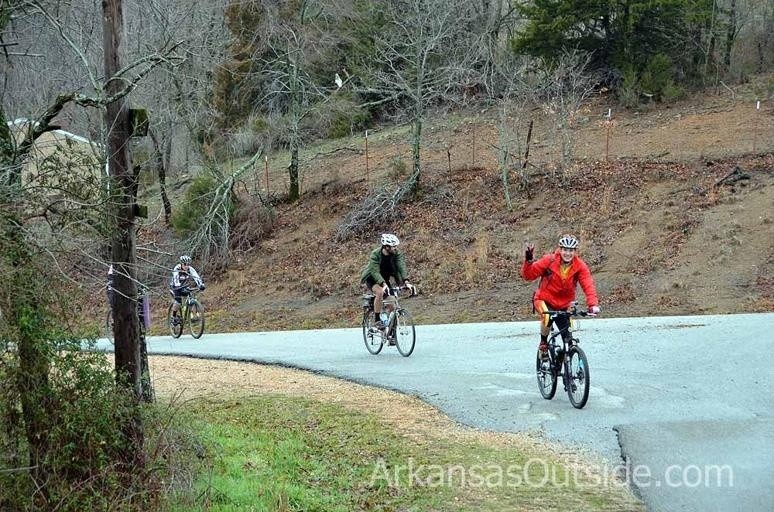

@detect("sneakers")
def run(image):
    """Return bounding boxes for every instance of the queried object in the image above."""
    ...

[564,378,577,390]
[375,321,385,329]
[539,342,547,359]
[389,337,395,345]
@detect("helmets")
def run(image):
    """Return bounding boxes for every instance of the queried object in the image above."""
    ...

[381,234,400,246]
[558,234,579,248]
[180,255,192,263]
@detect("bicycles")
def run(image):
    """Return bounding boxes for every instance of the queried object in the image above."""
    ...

[537,301,597,409]
[105,287,149,346]
[363,287,415,357]
[168,287,204,339]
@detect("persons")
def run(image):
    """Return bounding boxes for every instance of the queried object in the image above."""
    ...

[168,255,204,326]
[521,236,601,391]
[106,260,146,327]
[359,233,416,345]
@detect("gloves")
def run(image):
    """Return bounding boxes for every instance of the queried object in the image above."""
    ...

[525,246,534,261]
[589,305,600,313]
[382,285,390,295]
[200,285,205,290]
[407,283,417,296]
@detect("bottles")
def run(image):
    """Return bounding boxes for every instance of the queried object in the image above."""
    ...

[381,311,394,326]
